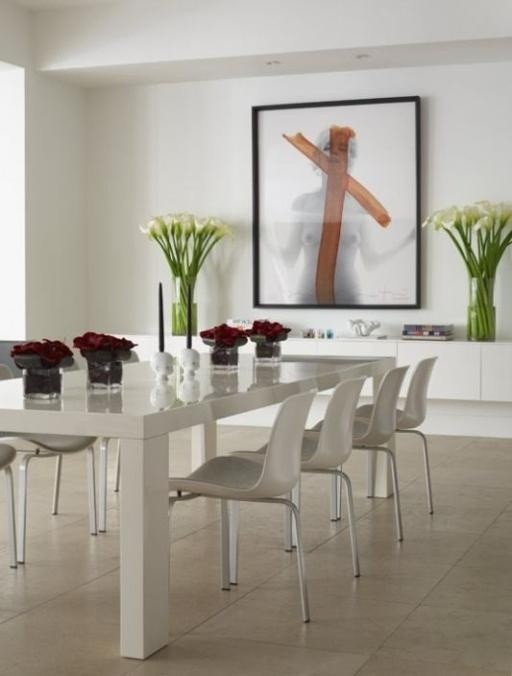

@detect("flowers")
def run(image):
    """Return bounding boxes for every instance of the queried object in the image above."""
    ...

[244,319,293,357]
[12,340,73,397]
[72,332,133,385]
[200,322,249,368]
[139,211,232,335]
[419,196,512,339]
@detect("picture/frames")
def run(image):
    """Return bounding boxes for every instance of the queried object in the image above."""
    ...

[254,342,284,364]
[249,95,423,311]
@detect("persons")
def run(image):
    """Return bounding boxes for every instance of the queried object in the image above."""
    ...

[260,126,418,301]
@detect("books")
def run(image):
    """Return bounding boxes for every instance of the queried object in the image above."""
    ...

[400,323,456,341]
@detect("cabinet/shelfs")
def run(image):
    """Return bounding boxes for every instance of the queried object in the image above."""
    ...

[111,333,510,442]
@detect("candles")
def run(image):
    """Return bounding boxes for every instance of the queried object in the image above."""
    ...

[158,281,165,353]
[186,283,193,348]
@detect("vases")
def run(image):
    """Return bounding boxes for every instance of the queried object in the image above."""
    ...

[16,355,72,401]
[82,351,139,392]
[467,277,498,343]
[206,337,240,370]
[172,275,197,338]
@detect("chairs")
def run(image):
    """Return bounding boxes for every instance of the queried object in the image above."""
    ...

[315,358,409,545]
[0,344,138,567]
[335,352,437,524]
[167,389,314,627]
[229,376,367,583]
